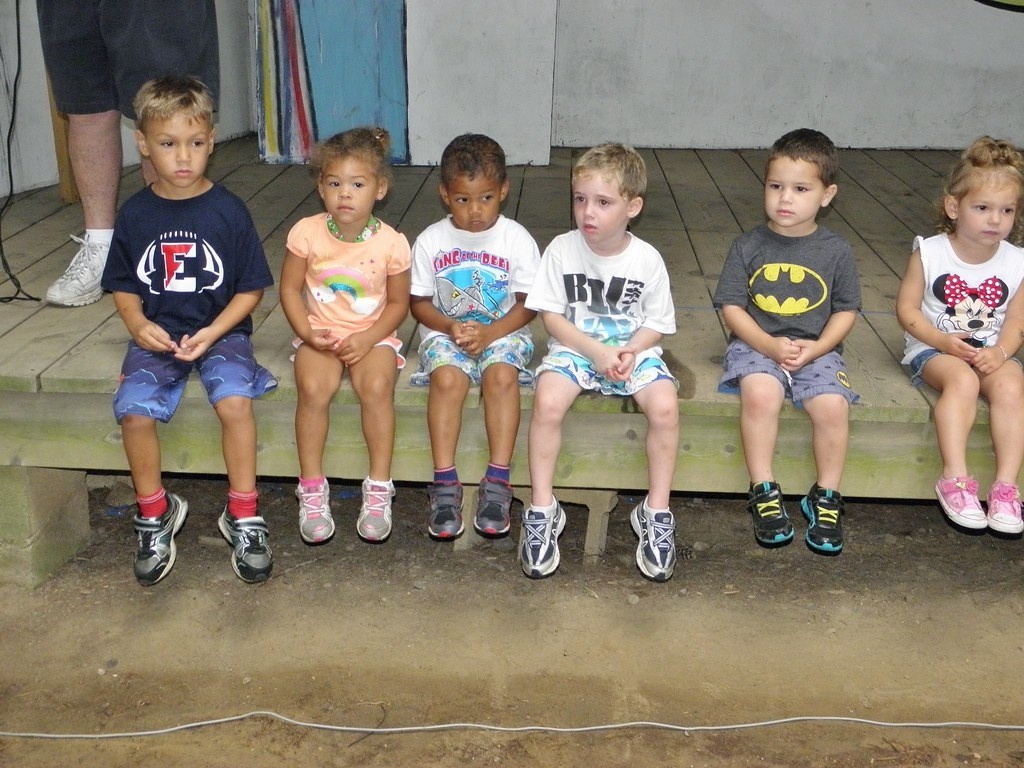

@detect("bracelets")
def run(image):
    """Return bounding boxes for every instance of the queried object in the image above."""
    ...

[992,343,1007,362]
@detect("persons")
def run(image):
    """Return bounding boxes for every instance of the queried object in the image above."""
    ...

[279,125,413,541]
[895,134,1024,534]
[520,140,682,581]
[410,133,542,539]
[713,128,864,554]
[36,0,219,307]
[99,69,279,588]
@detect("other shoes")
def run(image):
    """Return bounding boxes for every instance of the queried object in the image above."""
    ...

[987,480,1024,534]
[935,474,987,529]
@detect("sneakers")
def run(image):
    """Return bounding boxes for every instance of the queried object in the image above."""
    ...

[426,480,465,537]
[630,494,677,581]
[474,477,513,533]
[356,477,396,541]
[132,492,188,586]
[745,481,794,543]
[521,492,567,578]
[46,234,111,306]
[800,481,845,552]
[295,478,335,542]
[217,502,273,583]
